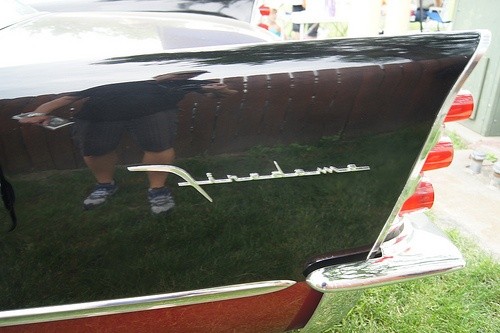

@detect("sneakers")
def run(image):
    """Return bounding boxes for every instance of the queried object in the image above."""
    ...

[83,178,121,208]
[147,184,175,216]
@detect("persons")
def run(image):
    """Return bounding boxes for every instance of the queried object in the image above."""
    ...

[423,1,454,32]
[268,8,282,37]
[18,69,236,216]
